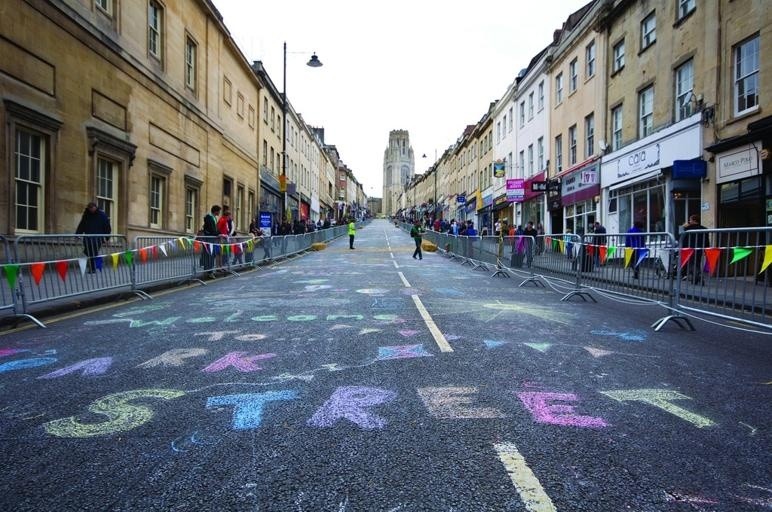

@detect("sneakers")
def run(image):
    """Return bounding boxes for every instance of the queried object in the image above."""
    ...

[691,275,705,286]
[682,274,687,281]
[412,255,423,260]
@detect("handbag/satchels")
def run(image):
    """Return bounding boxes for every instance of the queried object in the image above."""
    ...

[197,230,204,240]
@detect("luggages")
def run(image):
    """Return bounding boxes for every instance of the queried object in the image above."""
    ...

[583,254,595,272]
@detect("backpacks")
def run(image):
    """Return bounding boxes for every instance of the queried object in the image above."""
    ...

[410,231,414,237]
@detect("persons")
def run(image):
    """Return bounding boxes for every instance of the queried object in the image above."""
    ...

[75,202,111,273]
[626,220,645,279]
[594,222,606,268]
[388,214,544,262]
[200,205,371,278]
[566,229,574,259]
[679,215,709,287]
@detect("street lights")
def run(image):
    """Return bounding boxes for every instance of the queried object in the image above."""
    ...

[275,40,324,234]
[421,150,441,229]
